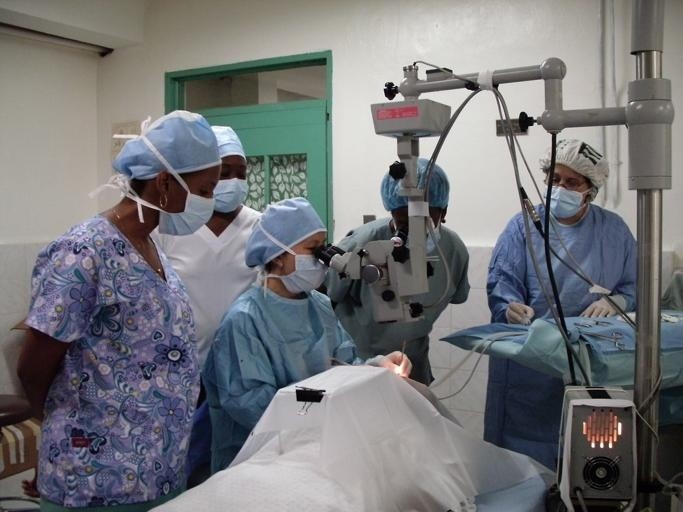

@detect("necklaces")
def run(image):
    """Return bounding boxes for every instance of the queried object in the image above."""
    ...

[111,205,163,278]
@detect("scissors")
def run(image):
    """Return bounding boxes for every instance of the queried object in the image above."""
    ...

[581,331,625,351]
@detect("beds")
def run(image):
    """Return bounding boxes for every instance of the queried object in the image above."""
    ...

[148,364,557,512]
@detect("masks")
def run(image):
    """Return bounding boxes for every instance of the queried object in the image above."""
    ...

[392,218,442,257]
[157,173,216,236]
[541,179,593,220]
[212,172,251,214]
[277,251,331,296]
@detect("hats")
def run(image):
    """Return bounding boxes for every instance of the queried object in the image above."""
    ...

[207,124,247,163]
[111,109,223,181]
[244,196,328,269]
[538,137,611,189]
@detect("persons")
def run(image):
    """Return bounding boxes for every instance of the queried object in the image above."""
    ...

[150,123,263,363]
[484,137,639,471]
[201,196,412,478]
[318,156,469,388]
[9,108,220,512]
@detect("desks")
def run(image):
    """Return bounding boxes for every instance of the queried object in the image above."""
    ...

[441,306,683,475]
